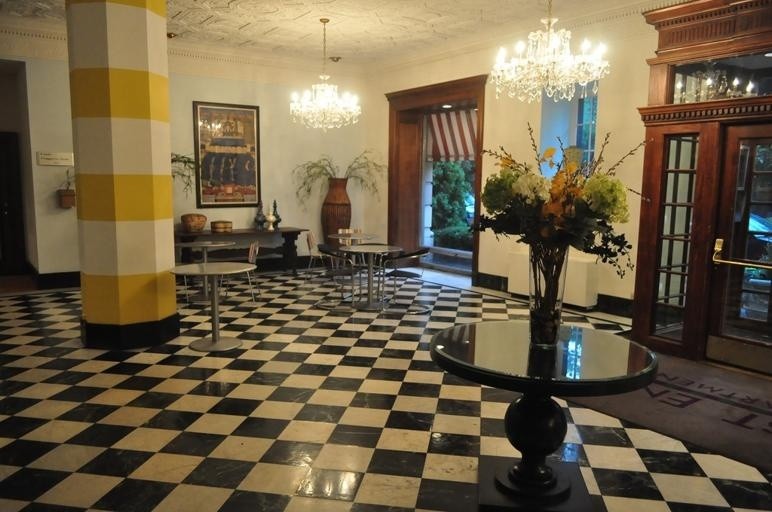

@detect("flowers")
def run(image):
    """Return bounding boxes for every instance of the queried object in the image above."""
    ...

[470,120,654,314]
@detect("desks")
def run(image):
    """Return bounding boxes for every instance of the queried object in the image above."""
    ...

[179,227,310,282]
[431,318,657,512]
[171,262,258,352]
[176,241,235,305]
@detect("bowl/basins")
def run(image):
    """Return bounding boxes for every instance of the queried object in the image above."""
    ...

[181,216,207,232]
[210,221,232,233]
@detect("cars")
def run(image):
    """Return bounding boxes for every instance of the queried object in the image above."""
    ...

[748,212,772,259]
[461,192,474,227]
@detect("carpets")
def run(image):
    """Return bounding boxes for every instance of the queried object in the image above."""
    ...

[555,349,772,474]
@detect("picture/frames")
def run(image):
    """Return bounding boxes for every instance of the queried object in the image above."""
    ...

[192,101,261,208]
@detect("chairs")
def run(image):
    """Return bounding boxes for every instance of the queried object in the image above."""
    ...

[176,261,202,303]
[219,241,261,303]
[306,228,432,316]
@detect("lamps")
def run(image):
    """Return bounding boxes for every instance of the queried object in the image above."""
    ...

[487,0,610,102]
[673,60,755,104]
[289,18,361,130]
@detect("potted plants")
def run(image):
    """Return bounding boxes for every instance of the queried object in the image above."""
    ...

[58,168,75,209]
[293,149,386,267]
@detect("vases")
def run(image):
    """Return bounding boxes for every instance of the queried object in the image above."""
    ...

[526,239,567,349]
